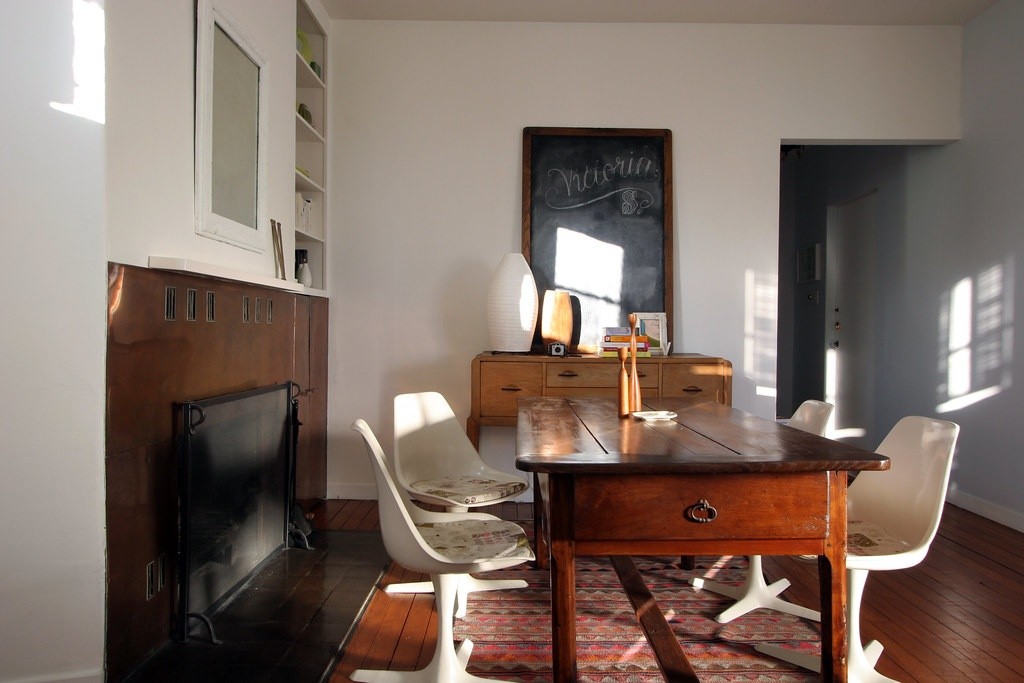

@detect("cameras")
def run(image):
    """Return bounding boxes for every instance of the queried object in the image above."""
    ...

[548,342,567,356]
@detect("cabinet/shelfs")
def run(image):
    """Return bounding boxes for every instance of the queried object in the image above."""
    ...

[105,0,333,297]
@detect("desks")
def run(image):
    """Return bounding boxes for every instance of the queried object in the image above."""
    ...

[515,396,890,683]
[465,353,734,453]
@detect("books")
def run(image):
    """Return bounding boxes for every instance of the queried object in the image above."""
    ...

[599,326,651,358]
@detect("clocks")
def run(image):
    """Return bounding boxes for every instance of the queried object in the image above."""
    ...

[795,243,822,284]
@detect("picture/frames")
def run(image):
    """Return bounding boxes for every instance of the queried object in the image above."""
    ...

[632,312,668,356]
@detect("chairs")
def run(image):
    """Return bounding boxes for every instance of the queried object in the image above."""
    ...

[383,391,530,596]
[754,416,959,682]
[348,419,537,683]
[688,399,834,624]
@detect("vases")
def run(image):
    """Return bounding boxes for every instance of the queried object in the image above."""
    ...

[542,291,572,351]
[483,253,539,352]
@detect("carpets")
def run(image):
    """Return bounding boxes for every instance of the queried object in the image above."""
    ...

[450,556,838,683]
